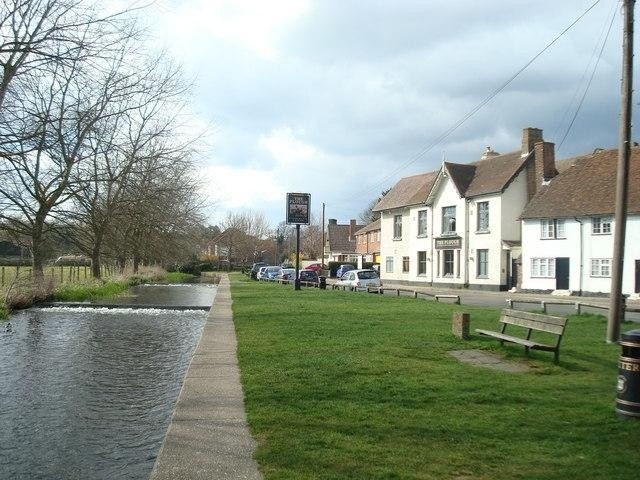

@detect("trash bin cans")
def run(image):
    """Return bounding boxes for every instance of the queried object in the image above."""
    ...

[615,328,640,417]
[319,277,326,290]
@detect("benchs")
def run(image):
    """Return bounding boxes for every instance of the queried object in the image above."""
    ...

[474,308,569,365]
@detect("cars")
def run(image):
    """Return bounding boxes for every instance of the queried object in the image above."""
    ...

[249,262,382,294]
